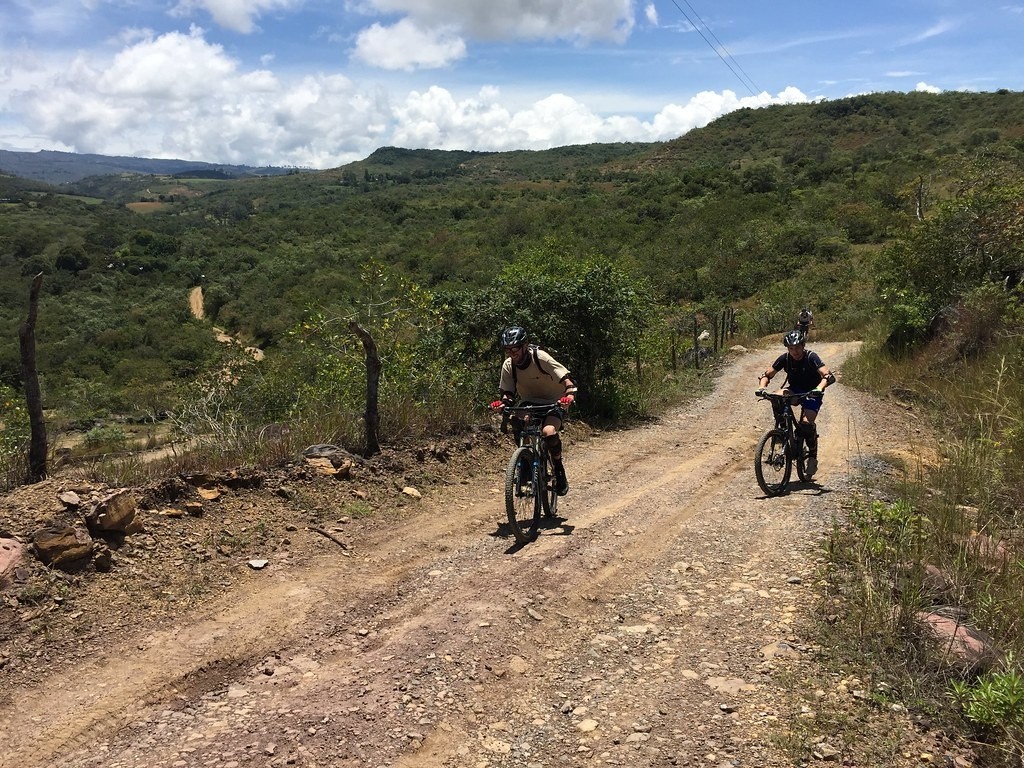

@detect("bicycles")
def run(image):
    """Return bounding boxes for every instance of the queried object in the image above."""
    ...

[797,324,813,342]
[487,400,578,545]
[754,390,824,497]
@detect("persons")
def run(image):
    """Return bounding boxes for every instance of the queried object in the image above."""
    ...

[797,307,813,337]
[754,329,837,476]
[491,325,577,496]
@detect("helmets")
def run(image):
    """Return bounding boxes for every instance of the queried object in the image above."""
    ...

[500,326,528,346]
[802,309,806,311]
[783,330,805,346]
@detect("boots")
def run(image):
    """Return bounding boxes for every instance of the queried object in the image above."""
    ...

[513,478,517,484]
[552,458,569,496]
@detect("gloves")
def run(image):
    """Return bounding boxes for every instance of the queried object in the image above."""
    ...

[491,401,502,412]
[755,387,766,396]
[560,397,573,408]
[808,386,824,398]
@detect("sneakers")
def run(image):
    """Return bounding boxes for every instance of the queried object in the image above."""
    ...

[805,458,818,475]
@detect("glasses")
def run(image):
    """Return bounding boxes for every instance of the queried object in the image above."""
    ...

[504,345,523,352]
